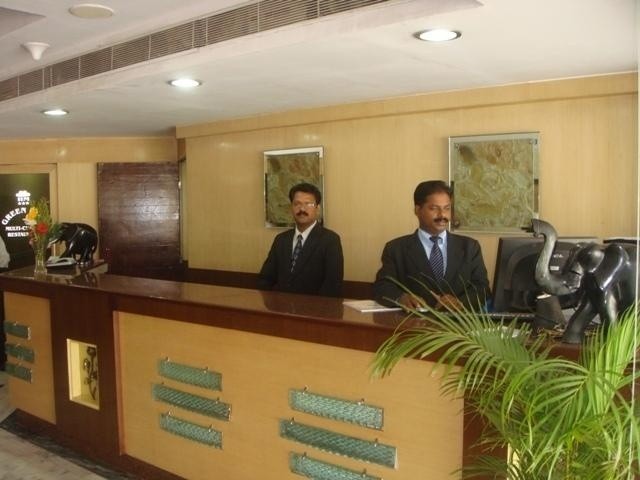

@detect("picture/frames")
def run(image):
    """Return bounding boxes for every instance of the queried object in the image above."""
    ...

[263,146,325,231]
[445,132,541,239]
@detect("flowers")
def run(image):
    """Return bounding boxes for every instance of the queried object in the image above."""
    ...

[25,196,64,268]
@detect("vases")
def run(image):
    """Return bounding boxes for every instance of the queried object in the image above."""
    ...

[33,240,48,274]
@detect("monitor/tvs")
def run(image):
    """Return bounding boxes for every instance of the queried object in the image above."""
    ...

[491,237,597,331]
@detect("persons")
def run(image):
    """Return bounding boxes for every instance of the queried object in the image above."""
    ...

[369,179,492,313]
[252,182,345,296]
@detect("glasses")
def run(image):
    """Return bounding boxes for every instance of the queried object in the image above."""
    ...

[291,201,318,208]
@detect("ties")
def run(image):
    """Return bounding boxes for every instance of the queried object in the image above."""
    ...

[428,236,444,285]
[290,235,303,275]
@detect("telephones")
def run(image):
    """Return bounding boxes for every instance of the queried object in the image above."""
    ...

[46,257,77,268]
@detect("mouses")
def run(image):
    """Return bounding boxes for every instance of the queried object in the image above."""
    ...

[415,306,429,313]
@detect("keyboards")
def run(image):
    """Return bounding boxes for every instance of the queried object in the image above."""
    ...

[436,310,535,322]
